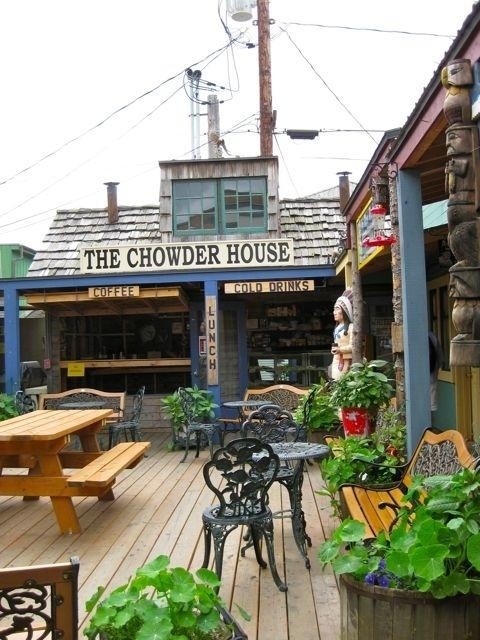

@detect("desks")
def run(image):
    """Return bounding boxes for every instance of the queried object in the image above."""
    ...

[240,441,333,571]
[0,401,114,535]
[222,400,274,446]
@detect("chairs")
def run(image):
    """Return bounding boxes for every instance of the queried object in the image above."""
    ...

[178,386,222,463]
[0,553,81,640]
[13,389,36,416]
[200,403,308,595]
[108,385,150,458]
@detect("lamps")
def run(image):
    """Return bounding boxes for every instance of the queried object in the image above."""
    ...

[362,203,399,247]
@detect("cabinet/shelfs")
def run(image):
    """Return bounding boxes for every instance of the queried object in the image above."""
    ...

[262,301,334,350]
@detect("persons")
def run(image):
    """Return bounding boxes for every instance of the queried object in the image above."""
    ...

[428,330,446,412]
[330,288,354,380]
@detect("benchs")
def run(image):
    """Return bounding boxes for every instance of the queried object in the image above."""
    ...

[338,424,478,551]
[65,440,152,497]
[213,383,317,450]
[38,387,127,435]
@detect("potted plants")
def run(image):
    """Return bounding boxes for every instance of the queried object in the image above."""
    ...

[325,356,397,439]
[318,466,479,640]
[158,383,221,451]
